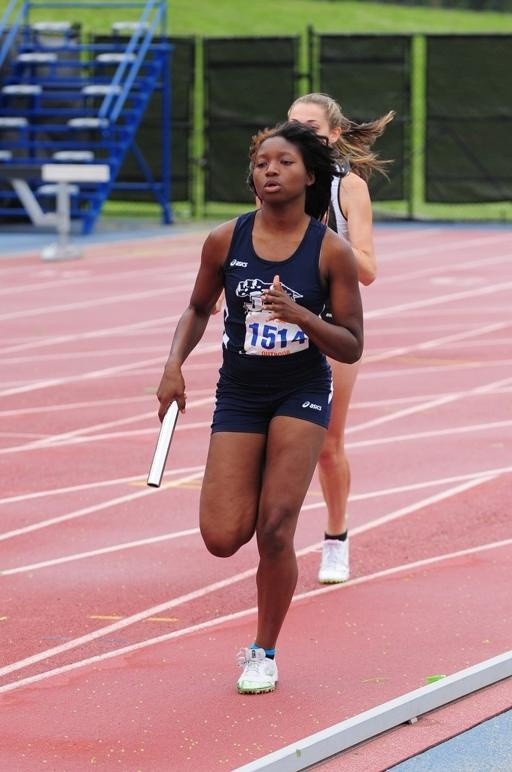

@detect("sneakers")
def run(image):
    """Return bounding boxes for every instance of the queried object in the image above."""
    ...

[235,646,279,695]
[317,527,350,584]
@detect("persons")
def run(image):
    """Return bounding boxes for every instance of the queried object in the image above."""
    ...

[153,118,367,694]
[252,89,399,589]
